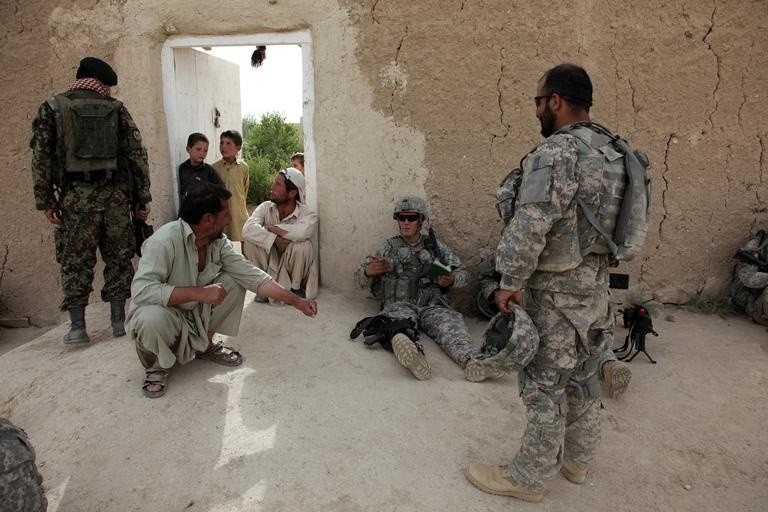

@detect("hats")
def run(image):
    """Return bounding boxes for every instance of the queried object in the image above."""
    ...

[75,57,118,88]
[281,167,306,204]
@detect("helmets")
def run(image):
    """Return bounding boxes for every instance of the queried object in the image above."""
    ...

[472,301,540,372]
[393,195,428,221]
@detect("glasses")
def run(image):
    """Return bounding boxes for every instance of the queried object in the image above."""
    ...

[534,94,568,109]
[398,214,420,222]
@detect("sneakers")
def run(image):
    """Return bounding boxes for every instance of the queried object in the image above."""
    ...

[603,360,632,398]
[391,334,432,381]
[465,462,544,502]
[465,359,510,381]
[560,457,588,485]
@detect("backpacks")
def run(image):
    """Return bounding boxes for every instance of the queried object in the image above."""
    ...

[51,94,124,183]
[556,122,652,262]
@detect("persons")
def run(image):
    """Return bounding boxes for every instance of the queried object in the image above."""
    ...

[241,166,319,306]
[212,130,250,255]
[0,417,49,512]
[122,183,316,398]
[463,63,650,501]
[29,57,153,344]
[478,256,632,398]
[291,153,305,176]
[353,196,489,382]
[177,133,223,215]
[734,229,768,326]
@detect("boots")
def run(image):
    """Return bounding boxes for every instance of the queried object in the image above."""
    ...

[109,299,126,337]
[63,306,89,344]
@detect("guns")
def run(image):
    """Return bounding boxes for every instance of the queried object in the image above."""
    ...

[613,305,658,363]
[424,228,449,294]
[133,203,153,257]
[730,246,768,273]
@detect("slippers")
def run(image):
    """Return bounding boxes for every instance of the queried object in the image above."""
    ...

[194,341,243,367]
[141,367,169,397]
